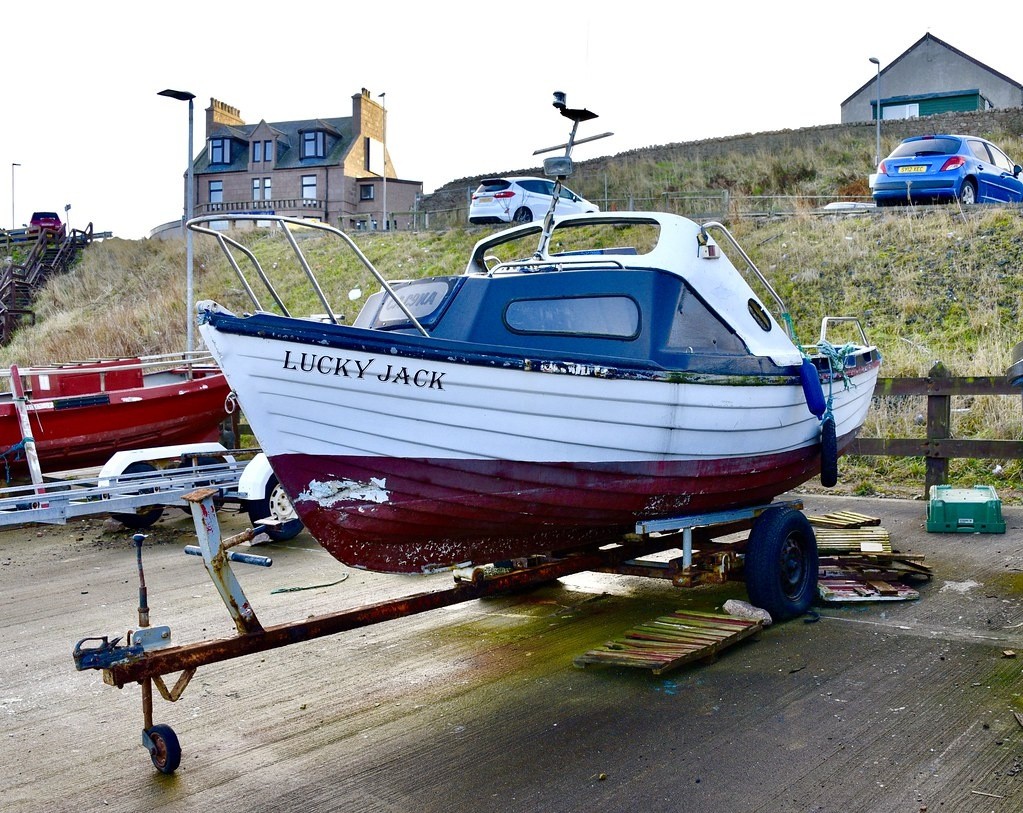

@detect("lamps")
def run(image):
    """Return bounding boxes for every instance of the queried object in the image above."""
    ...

[543,157,573,180]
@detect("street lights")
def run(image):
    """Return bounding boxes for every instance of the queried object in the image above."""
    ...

[157,87,196,370]
[65,205,71,234]
[869,56,880,167]
[11,162,22,231]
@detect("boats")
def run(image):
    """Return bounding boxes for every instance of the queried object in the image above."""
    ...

[181,91,882,577]
[0,352,242,473]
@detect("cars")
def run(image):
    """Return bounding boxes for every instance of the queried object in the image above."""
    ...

[872,134,1023,208]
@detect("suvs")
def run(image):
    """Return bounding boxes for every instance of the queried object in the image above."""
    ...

[468,176,600,225]
[23,211,65,241]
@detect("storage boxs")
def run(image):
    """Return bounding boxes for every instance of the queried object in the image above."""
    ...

[925,485,1006,533]
